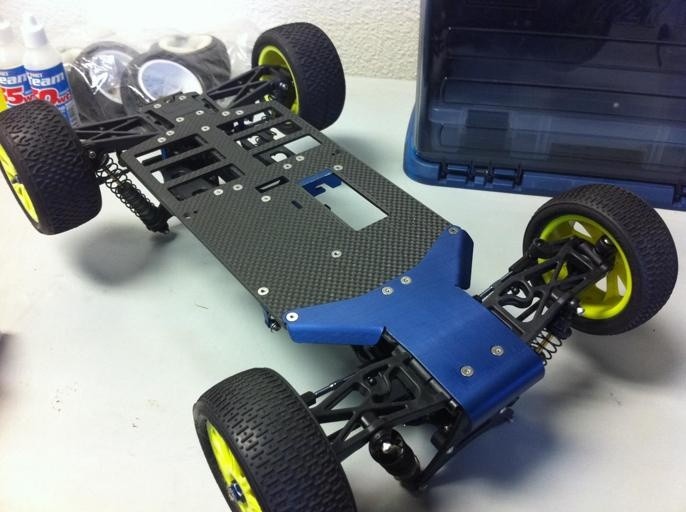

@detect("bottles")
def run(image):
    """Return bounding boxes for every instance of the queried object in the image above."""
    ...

[0,11,82,130]
